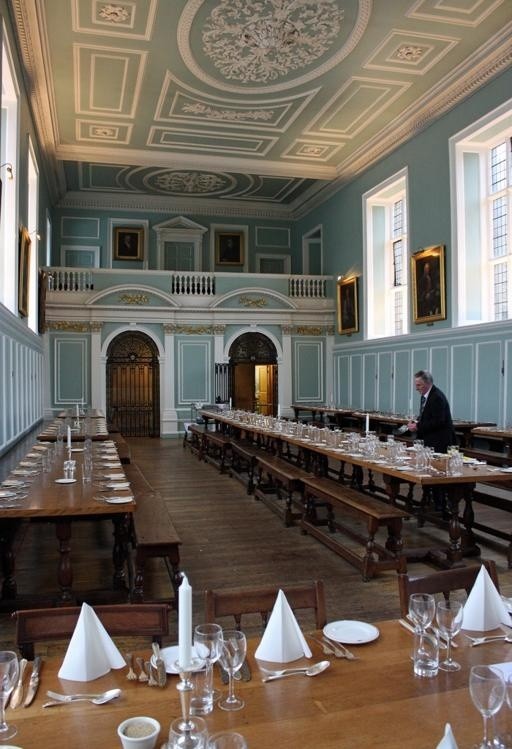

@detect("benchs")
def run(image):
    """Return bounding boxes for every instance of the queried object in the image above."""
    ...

[130,491,183,602]
[107,432,131,464]
[458,446,512,467]
[107,420,119,432]
[120,463,154,495]
[382,435,414,446]
[254,454,314,528]
[300,474,412,583]
[229,439,266,495]
[182,424,211,459]
[202,430,237,475]
[418,477,512,570]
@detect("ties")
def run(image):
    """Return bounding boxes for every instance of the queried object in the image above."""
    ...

[421,397,425,413]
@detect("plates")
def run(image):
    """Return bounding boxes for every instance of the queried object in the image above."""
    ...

[11,470,39,477]
[32,445,48,452]
[104,473,126,478]
[0,489,17,501]
[19,461,37,467]
[55,478,77,484]
[104,482,131,488]
[69,448,84,452]
[102,448,118,454]
[101,455,119,461]
[100,462,123,467]
[2,481,25,486]
[149,644,208,673]
[25,453,42,459]
[106,496,132,503]
[322,621,380,645]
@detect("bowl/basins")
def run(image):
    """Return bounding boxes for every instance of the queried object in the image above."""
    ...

[117,716,162,748]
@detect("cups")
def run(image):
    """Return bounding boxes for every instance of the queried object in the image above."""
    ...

[54,442,62,456]
[47,449,54,465]
[82,439,94,481]
[57,435,64,449]
[187,658,215,715]
[414,635,440,677]
[42,457,51,472]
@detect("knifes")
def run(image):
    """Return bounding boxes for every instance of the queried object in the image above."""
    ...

[234,671,241,681]
[397,618,449,649]
[151,642,166,687]
[24,656,42,706]
[229,635,251,682]
[241,661,250,681]
[11,657,26,709]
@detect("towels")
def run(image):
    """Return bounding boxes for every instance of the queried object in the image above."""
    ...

[57,602,127,682]
[461,564,512,630]
[254,589,312,663]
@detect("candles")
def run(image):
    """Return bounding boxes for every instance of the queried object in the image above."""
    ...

[177,570,194,675]
[65,424,72,460]
[277,403,280,415]
[82,397,83,407]
[76,404,80,415]
[229,397,232,409]
[365,412,370,431]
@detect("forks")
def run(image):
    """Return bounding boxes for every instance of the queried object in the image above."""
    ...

[322,637,346,657]
[306,633,335,656]
[136,657,149,682]
[144,661,159,688]
[466,634,507,640]
[335,641,360,662]
[258,666,310,675]
[127,653,137,680]
[45,690,105,702]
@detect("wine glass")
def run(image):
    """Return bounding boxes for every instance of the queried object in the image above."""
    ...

[218,629,247,711]
[215,404,465,470]
[469,665,498,749]
[489,671,512,749]
[168,717,208,749]
[206,731,248,749]
[195,623,223,701]
[0,652,18,741]
[438,600,464,673]
[408,592,436,663]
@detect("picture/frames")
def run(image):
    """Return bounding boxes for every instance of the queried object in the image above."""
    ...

[215,228,245,267]
[410,245,445,324]
[112,225,145,261]
[335,277,358,335]
[19,227,32,318]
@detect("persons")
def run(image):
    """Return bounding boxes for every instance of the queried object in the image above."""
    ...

[120,235,137,257]
[343,289,354,327]
[407,370,454,512]
[418,262,438,315]
[222,239,239,263]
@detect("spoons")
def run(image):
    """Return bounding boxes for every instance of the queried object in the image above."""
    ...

[42,688,122,709]
[261,660,331,682]
[469,634,512,648]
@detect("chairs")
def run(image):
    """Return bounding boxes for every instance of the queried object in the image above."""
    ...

[10,605,171,662]
[203,583,327,634]
